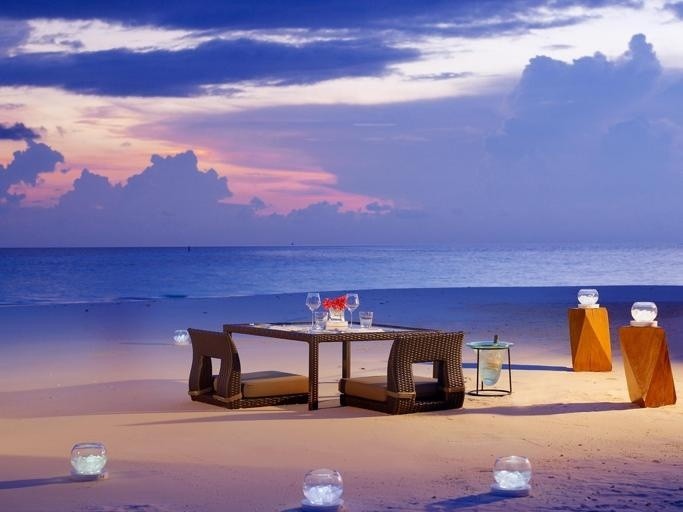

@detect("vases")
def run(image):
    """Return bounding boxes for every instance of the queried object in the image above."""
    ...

[329,312,345,321]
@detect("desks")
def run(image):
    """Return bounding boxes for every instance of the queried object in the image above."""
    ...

[222,318,444,412]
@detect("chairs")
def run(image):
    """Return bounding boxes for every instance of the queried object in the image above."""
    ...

[337,331,465,415]
[184,328,308,410]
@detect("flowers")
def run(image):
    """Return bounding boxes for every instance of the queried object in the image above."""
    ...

[322,295,347,311]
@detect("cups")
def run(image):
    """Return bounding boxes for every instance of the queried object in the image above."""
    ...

[494,455,532,489]
[314,312,328,328]
[358,312,373,329]
[69,444,106,474]
[629,300,660,322]
[577,288,599,306]
[172,328,191,343]
[301,466,343,504]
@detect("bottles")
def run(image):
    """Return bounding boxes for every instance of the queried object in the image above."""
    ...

[483,334,501,383]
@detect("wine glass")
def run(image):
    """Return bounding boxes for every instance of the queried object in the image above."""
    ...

[343,293,358,328]
[304,292,321,331]
